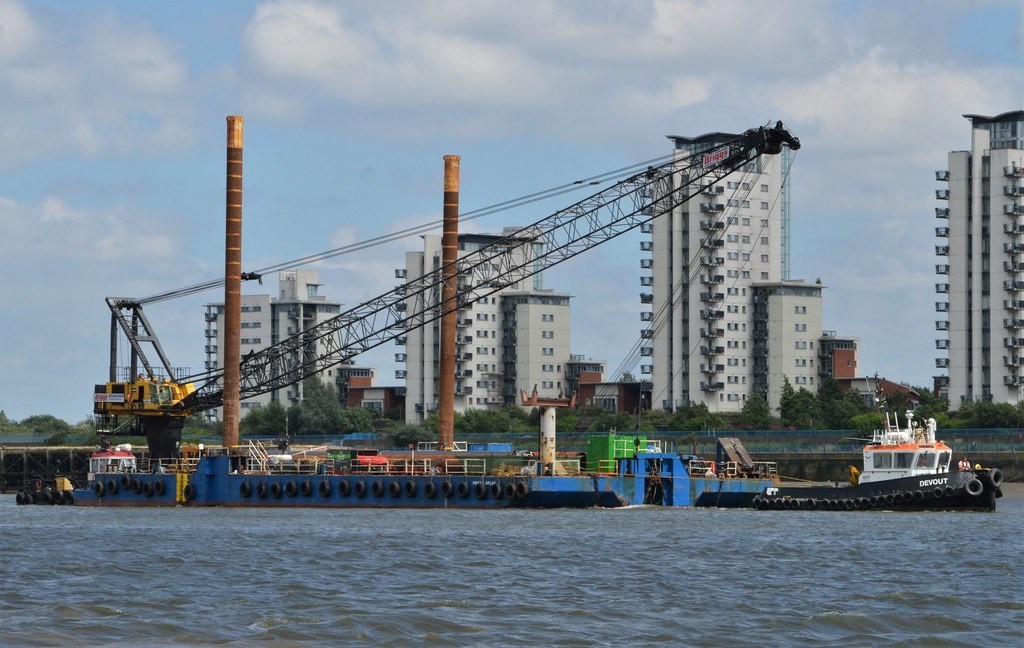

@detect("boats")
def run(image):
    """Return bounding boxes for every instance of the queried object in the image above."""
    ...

[65,384,783,508]
[774,408,1004,513]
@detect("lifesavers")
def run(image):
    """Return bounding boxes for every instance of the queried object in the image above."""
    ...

[404,480,417,497]
[338,479,351,497]
[388,481,401,498]
[93,480,105,497]
[974,463,982,469]
[319,480,331,497]
[752,485,956,510]
[269,482,283,499]
[299,480,312,497]
[131,478,142,495]
[474,482,486,499]
[441,480,454,497]
[154,479,166,495]
[354,480,366,498]
[285,481,298,498]
[106,478,119,495]
[239,481,251,497]
[256,485,267,498]
[987,468,1004,489]
[436,466,442,474]
[504,481,528,501]
[143,481,153,497]
[423,481,436,499]
[491,483,503,499]
[127,393,133,404]
[15,489,75,506]
[371,480,384,498]
[121,475,132,490]
[964,478,984,498]
[458,481,469,498]
[184,484,195,500]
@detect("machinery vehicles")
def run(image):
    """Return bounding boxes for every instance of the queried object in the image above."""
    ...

[92,118,802,456]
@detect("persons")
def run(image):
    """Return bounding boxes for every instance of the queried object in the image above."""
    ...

[958,456,971,472]
